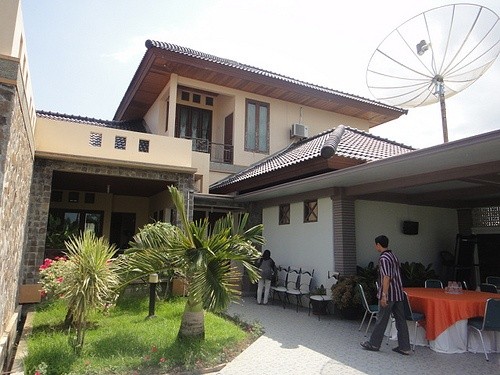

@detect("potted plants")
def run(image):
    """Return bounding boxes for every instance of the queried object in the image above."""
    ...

[305,283,327,316]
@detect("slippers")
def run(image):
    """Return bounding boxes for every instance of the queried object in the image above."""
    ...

[392,347,409,355]
[360,341,379,351]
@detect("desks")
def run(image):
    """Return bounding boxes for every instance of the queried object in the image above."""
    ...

[385,287,500,353]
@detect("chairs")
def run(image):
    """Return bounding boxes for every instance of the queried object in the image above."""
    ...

[358,276,500,362]
[256,264,338,321]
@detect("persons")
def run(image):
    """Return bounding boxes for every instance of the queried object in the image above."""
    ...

[359,235,411,355]
[255,250,277,305]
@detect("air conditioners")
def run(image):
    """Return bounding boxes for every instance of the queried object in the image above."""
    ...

[290,123,311,140]
